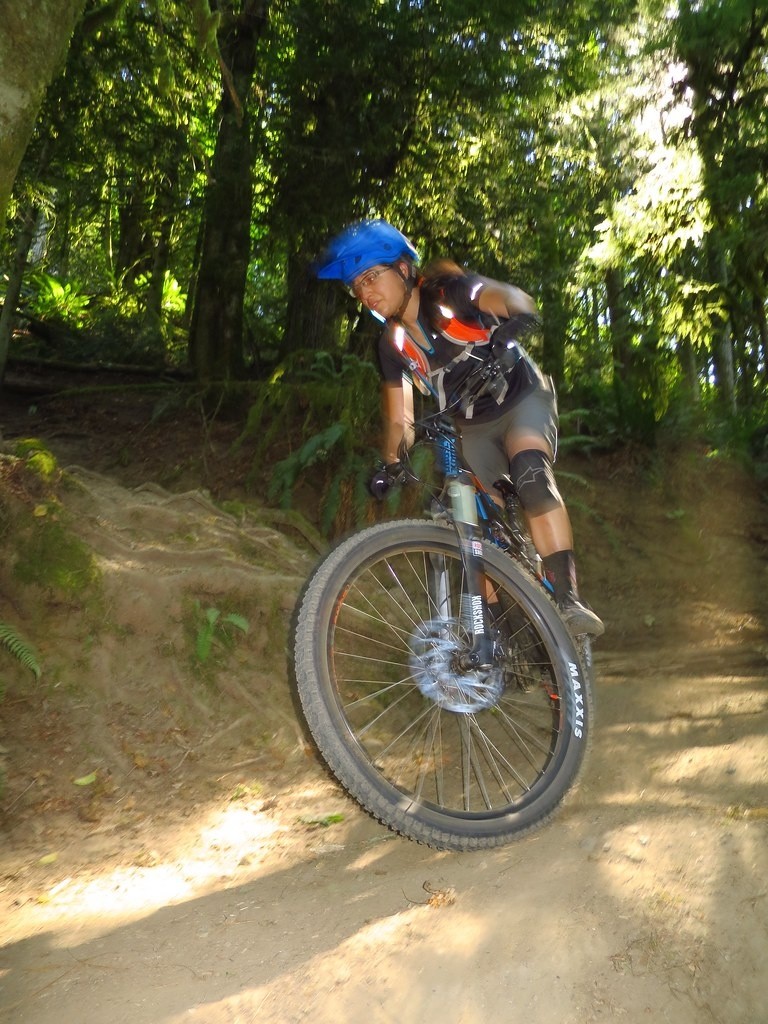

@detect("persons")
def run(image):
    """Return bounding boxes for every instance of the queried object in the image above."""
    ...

[316,220,606,642]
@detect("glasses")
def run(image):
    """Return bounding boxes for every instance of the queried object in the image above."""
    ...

[348,265,395,298]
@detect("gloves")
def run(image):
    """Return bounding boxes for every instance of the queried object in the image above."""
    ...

[370,461,405,499]
[489,313,541,367]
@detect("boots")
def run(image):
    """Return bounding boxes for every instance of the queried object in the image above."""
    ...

[485,602,516,661]
[541,549,605,643]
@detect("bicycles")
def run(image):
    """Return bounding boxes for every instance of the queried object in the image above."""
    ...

[293,345,607,852]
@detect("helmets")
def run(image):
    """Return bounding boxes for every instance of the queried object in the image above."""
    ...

[316,220,420,286]
